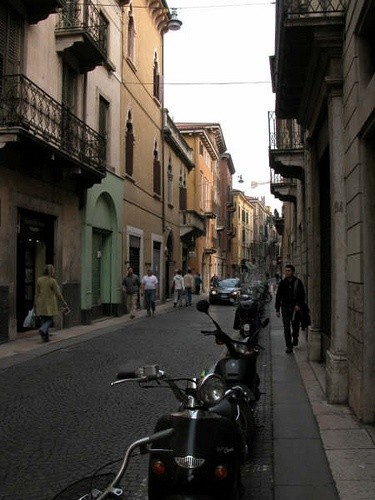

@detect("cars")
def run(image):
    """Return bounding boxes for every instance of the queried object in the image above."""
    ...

[210,277,239,302]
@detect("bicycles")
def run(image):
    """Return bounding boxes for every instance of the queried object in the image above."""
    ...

[50,428,174,500]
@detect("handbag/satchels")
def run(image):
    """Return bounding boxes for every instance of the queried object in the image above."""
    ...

[292,303,309,321]
[23,308,35,327]
[31,316,41,328]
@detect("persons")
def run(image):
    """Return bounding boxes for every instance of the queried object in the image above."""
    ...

[33,264,71,342]
[141,269,158,317]
[210,274,219,286]
[123,267,141,318]
[171,267,202,307]
[275,264,306,354]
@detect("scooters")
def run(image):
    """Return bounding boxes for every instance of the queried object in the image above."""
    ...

[196,300,271,400]
[232,284,274,340]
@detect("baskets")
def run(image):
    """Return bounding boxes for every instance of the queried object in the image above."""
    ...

[51,473,116,500]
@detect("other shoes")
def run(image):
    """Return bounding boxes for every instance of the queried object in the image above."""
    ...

[146,310,150,317]
[293,337,298,346]
[286,348,292,353]
[39,330,47,342]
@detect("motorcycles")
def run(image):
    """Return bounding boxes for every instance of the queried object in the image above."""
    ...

[112,364,256,500]
